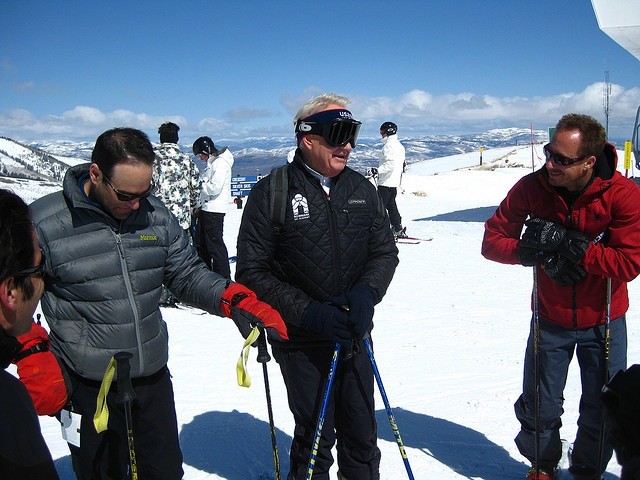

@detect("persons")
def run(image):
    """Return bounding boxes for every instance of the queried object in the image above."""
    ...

[10,127,289,480]
[234,94,399,480]
[151,122,201,308]
[192,136,234,280]
[368,122,407,242]
[0,189,59,480]
[481,113,640,480]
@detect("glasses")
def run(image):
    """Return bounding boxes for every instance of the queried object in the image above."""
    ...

[294,117,363,149]
[197,152,204,159]
[544,142,594,170]
[104,177,155,202]
[380,130,386,135]
[13,242,50,286]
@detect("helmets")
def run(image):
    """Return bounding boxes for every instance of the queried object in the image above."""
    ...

[380,122,398,137]
[192,136,214,156]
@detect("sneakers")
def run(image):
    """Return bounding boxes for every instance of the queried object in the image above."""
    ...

[391,227,403,237]
[525,470,555,480]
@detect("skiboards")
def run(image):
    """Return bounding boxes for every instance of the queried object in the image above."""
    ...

[395,235,433,245]
[174,302,206,316]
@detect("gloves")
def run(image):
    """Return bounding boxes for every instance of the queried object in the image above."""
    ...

[299,301,354,349]
[518,218,590,263]
[221,284,289,347]
[515,239,587,288]
[10,323,69,416]
[330,282,375,340]
